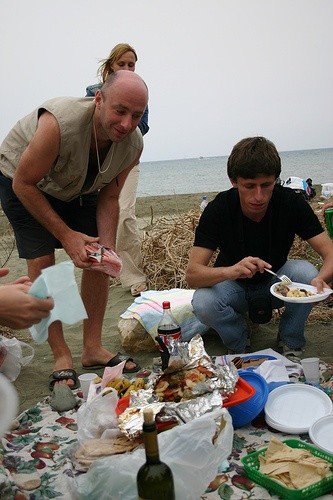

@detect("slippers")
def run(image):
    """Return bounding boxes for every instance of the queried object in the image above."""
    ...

[80,347,140,374]
[46,357,79,392]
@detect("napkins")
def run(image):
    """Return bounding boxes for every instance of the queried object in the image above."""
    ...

[255,358,292,384]
[30,261,89,340]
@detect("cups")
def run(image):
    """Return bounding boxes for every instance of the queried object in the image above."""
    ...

[77,373,99,399]
[300,357,320,384]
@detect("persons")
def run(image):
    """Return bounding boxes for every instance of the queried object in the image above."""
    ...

[302,178,316,201]
[0,70,148,390]
[0,268,54,330]
[185,137,333,363]
[86,44,149,297]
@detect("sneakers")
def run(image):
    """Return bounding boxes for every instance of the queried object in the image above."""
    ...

[277,332,304,359]
[229,338,250,354]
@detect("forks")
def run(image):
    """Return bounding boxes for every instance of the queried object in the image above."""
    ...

[86,246,104,264]
[264,267,292,283]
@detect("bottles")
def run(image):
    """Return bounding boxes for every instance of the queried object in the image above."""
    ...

[137,408,175,500]
[157,301,182,370]
[201,196,208,212]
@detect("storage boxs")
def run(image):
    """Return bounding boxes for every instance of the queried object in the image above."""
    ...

[243,440,333,500]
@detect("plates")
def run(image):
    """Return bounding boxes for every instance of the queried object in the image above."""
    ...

[228,372,269,427]
[309,415,333,455]
[238,355,277,371]
[270,282,333,303]
[264,384,333,434]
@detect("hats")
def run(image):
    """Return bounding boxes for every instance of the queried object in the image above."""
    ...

[283,176,306,190]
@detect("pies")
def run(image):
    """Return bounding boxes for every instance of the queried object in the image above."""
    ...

[82,439,140,456]
[258,440,332,491]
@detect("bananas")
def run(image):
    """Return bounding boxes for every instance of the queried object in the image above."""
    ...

[103,379,144,398]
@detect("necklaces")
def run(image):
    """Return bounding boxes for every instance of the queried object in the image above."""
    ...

[92,117,115,174]
